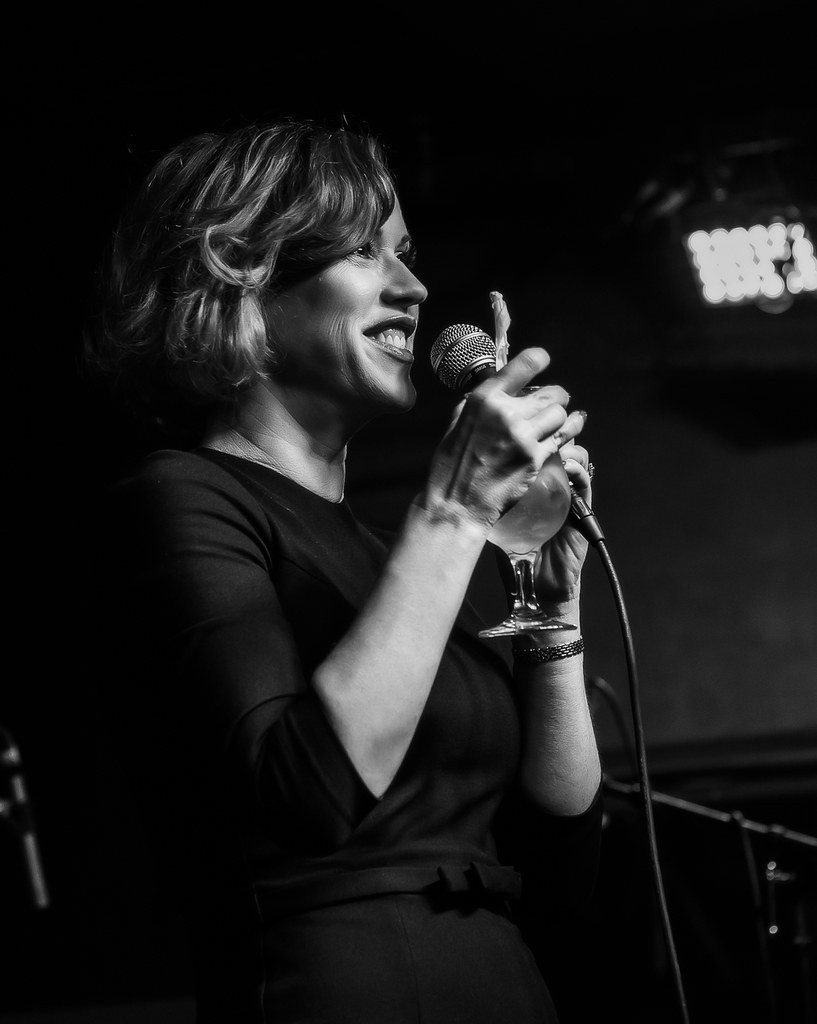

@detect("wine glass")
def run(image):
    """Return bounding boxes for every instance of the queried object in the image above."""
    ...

[477,452,577,638]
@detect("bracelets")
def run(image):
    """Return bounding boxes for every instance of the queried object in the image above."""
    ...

[531,637,584,662]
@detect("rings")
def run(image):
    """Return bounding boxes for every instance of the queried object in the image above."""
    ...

[588,462,594,479]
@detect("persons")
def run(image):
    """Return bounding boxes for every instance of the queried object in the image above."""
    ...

[114,125,602,1024]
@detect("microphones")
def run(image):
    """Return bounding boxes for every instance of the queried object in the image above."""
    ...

[431,323,606,546]
[0,728,50,908]
